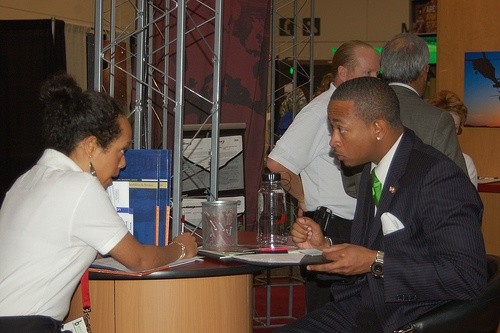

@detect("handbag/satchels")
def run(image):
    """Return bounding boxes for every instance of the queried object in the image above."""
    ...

[60,314,91,332]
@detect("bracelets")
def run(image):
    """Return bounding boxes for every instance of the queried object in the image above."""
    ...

[168,241,185,261]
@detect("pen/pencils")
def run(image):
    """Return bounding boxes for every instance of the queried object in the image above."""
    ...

[478,176,499,180]
[181,213,185,235]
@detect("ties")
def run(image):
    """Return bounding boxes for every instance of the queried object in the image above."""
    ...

[370,167,383,210]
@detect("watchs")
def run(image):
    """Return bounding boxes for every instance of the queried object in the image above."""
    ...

[371,250,384,280]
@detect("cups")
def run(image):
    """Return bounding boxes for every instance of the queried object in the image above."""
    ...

[201,200,240,249]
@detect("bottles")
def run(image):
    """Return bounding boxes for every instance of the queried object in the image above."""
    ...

[257,173,289,248]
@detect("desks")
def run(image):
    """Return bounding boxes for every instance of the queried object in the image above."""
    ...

[63,228,276,332]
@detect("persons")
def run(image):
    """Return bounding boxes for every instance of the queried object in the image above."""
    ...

[427,91,478,191]
[278,76,489,333]
[379,31,469,178]
[0,74,199,333]
[265,39,377,314]
